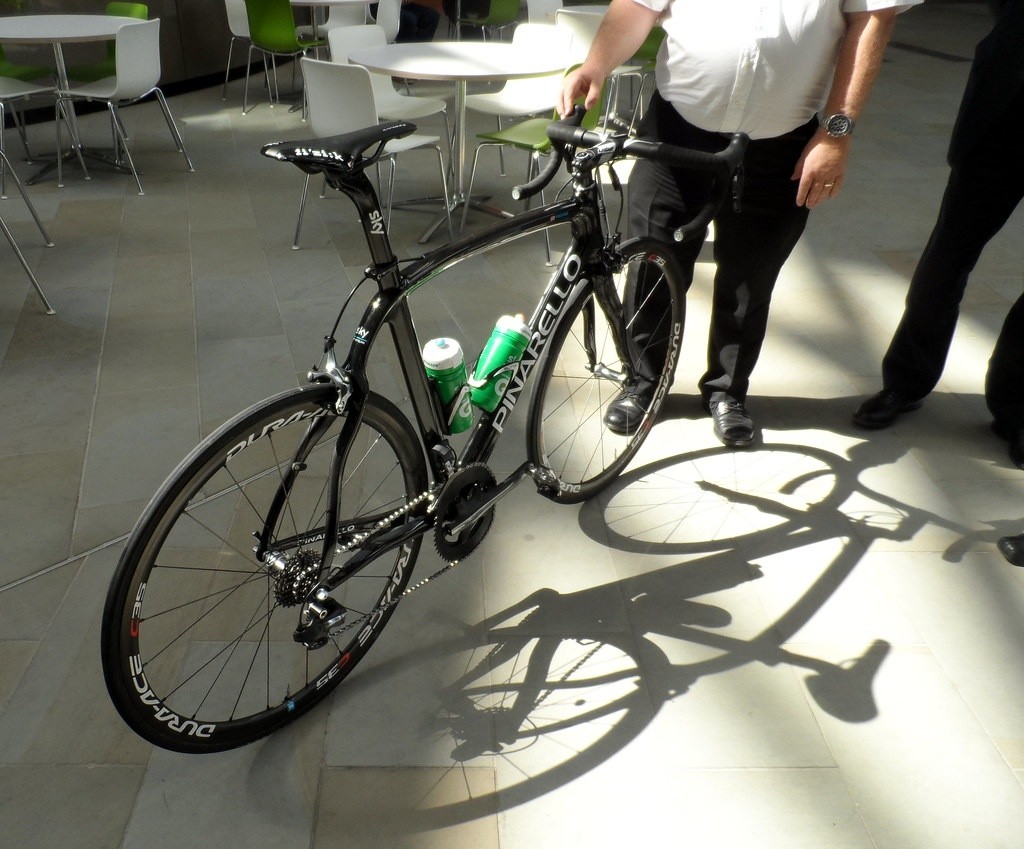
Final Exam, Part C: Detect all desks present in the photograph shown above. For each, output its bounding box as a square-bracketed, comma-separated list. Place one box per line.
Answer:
[0, 15, 149, 187]
[348, 42, 572, 243]
[563, 5, 611, 14]
[288, 0, 375, 113]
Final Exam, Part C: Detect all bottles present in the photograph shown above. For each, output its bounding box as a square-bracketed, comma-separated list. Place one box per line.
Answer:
[423, 337, 474, 434]
[469, 314, 532, 413]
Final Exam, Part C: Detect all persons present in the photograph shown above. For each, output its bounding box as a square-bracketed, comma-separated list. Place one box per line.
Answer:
[556, 0, 926, 448]
[850, 0, 1024, 469]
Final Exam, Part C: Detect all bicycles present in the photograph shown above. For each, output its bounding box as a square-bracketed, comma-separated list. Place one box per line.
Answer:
[97, 104, 752, 756]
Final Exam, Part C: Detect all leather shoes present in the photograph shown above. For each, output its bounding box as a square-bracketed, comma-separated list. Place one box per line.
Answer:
[603, 390, 648, 434]
[990, 420, 1024, 469]
[852, 390, 923, 431]
[997, 532, 1024, 567]
[708, 400, 755, 447]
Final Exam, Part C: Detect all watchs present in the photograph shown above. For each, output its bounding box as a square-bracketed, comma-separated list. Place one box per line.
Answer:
[816, 112, 856, 138]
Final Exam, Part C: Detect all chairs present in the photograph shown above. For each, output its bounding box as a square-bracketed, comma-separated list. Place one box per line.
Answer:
[459, 62, 612, 267]
[242, 0, 330, 122]
[61, 2, 184, 162]
[443, 20, 604, 222]
[0, 76, 91, 200]
[0, 44, 81, 164]
[0, 102, 56, 314]
[376, 1, 410, 97]
[222, 0, 295, 109]
[448, 0, 519, 42]
[291, 55, 455, 251]
[294, 0, 367, 60]
[53, 17, 195, 196]
[320, 25, 459, 229]
[367, 0, 376, 25]
[552, 5, 667, 139]
